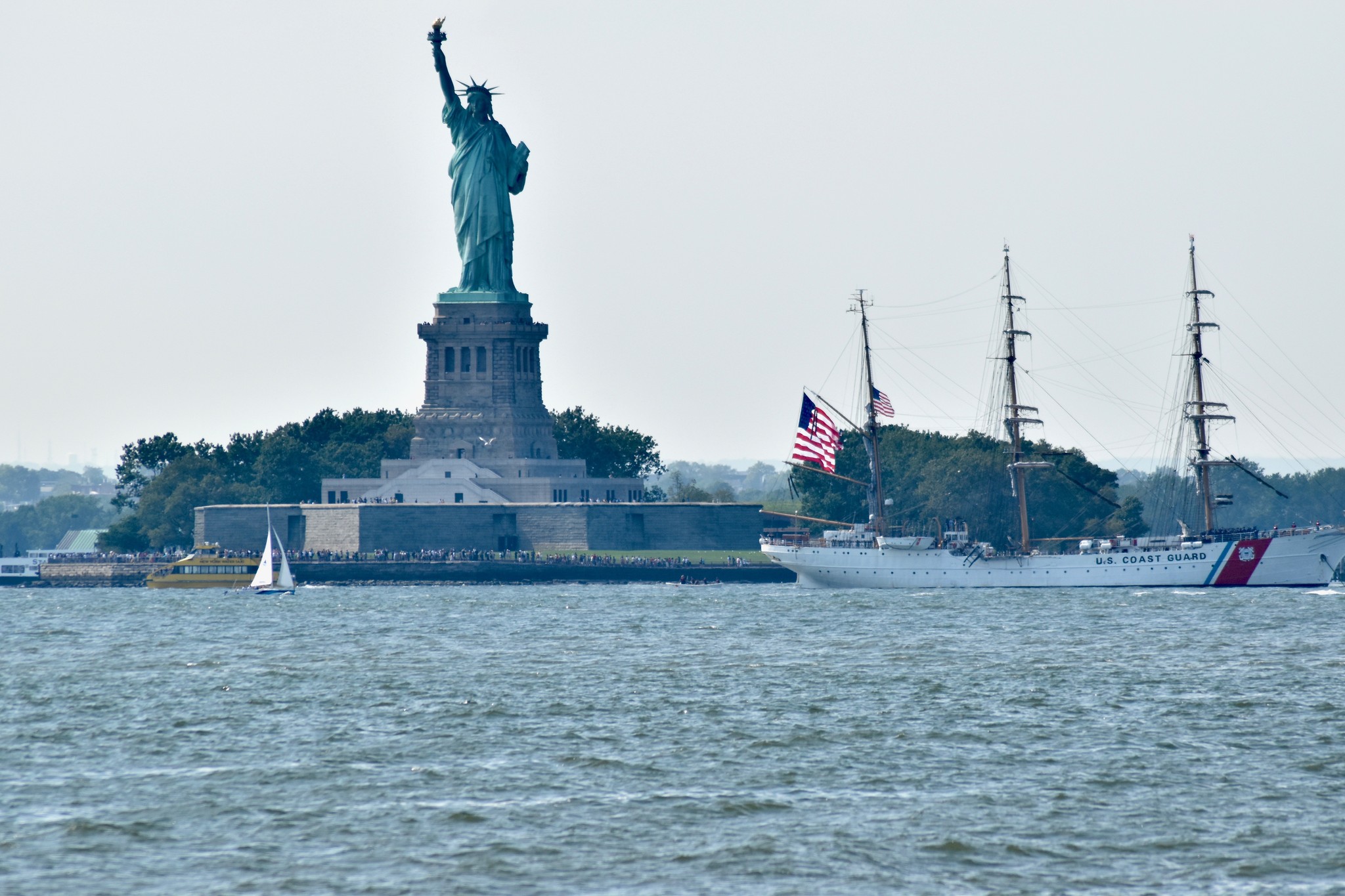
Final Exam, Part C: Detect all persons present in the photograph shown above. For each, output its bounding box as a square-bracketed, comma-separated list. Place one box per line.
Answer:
[759, 533, 774, 546]
[235, 584, 265, 591]
[680, 575, 720, 585]
[1199, 526, 1266, 543]
[1291, 522, 1297, 536]
[430, 42, 528, 292]
[49, 550, 186, 563]
[1273, 525, 1278, 537]
[1316, 521, 1320, 531]
[727, 555, 751, 567]
[942, 516, 973, 555]
[220, 548, 705, 567]
[579, 496, 648, 502]
[298, 496, 463, 505]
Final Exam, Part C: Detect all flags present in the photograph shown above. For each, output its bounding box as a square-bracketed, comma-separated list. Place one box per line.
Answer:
[792, 391, 847, 475]
[872, 386, 895, 418]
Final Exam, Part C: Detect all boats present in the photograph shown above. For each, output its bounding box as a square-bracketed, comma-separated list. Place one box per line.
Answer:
[876, 535, 935, 550]
[142, 541, 299, 590]
[665, 582, 724, 589]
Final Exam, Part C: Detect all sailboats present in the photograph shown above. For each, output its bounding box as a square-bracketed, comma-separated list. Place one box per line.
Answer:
[758, 232, 1345, 590]
[249, 500, 296, 596]
